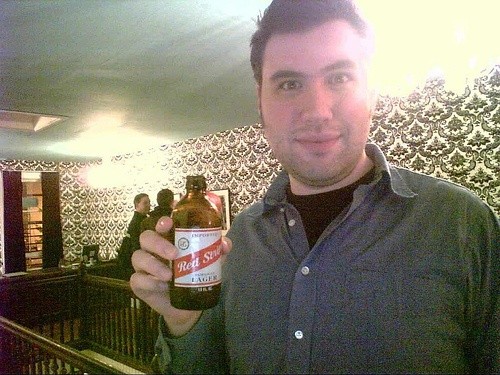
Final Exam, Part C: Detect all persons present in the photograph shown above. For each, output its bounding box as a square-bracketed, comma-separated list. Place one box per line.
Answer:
[118, 193, 152, 280]
[126, 0, 500, 374]
[139, 188, 176, 245]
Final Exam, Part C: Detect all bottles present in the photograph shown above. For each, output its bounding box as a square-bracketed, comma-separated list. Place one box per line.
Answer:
[167, 175, 223, 310]
